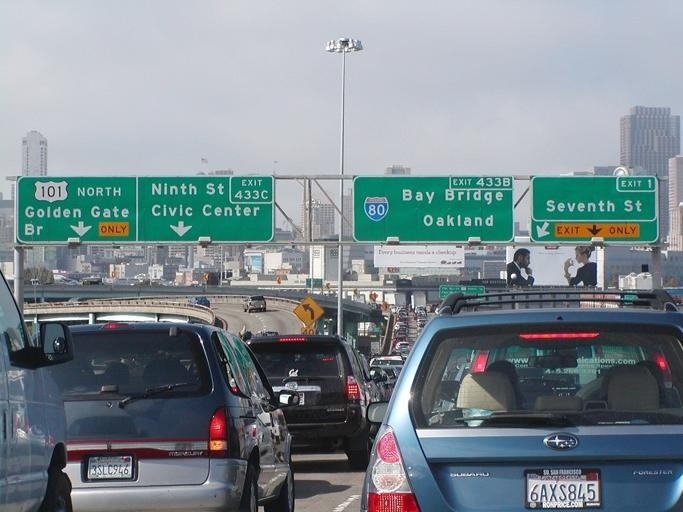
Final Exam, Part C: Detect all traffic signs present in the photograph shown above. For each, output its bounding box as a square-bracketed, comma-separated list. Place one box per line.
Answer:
[137, 177, 274, 243]
[531, 177, 658, 242]
[355, 178, 514, 244]
[17, 177, 134, 241]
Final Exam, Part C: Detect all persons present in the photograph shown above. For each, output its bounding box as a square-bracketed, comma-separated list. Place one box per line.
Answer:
[562, 245, 596, 288]
[506, 247, 535, 288]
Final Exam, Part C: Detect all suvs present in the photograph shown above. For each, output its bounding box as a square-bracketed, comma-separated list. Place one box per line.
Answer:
[240, 294, 266, 312]
[468, 344, 607, 431]
[241, 327, 388, 466]
[0, 266, 72, 511]
[361, 286, 682, 511]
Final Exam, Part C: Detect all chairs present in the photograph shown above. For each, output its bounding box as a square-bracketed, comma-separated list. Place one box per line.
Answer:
[607, 363, 660, 412]
[454, 372, 515, 410]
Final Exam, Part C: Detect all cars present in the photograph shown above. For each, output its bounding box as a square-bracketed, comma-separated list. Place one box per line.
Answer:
[187, 296, 210, 307]
[368, 303, 444, 389]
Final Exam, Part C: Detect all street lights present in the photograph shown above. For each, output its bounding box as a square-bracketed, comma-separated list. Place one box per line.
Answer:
[27, 274, 41, 346]
[325, 36, 366, 336]
[303, 199, 321, 289]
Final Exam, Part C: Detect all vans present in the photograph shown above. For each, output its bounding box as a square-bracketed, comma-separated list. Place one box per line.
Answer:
[55, 320, 294, 512]
